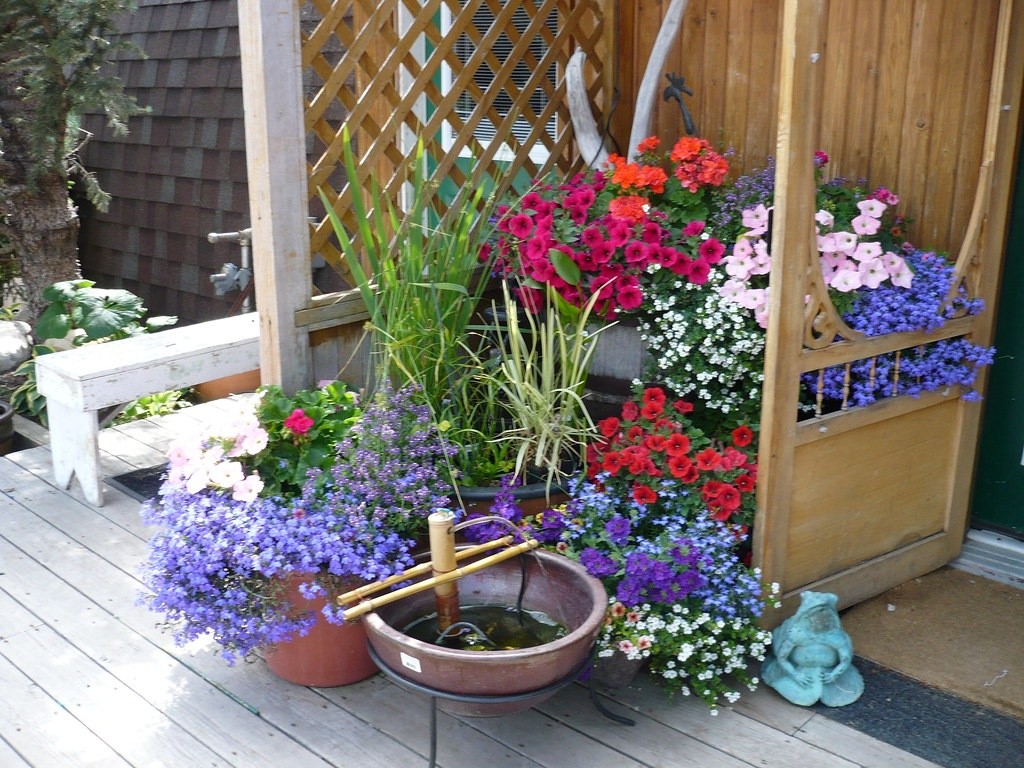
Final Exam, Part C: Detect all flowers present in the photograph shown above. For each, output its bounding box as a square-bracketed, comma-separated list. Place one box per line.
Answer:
[137, 122, 999, 718]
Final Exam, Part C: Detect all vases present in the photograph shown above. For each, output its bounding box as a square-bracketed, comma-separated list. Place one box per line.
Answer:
[261, 573, 382, 687]
[406, 443, 594, 554]
[362, 543, 609, 720]
[0, 399, 17, 457]
